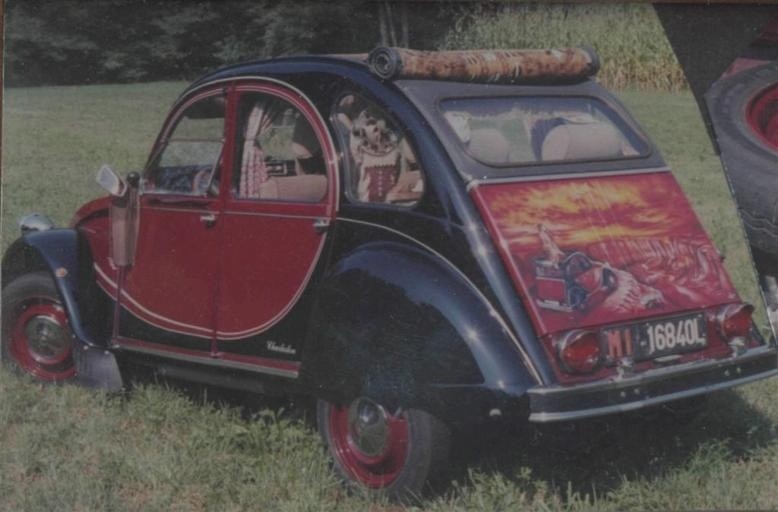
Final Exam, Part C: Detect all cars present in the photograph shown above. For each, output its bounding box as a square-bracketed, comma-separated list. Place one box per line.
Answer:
[0, 48, 778, 509]
[531, 249, 617, 314]
